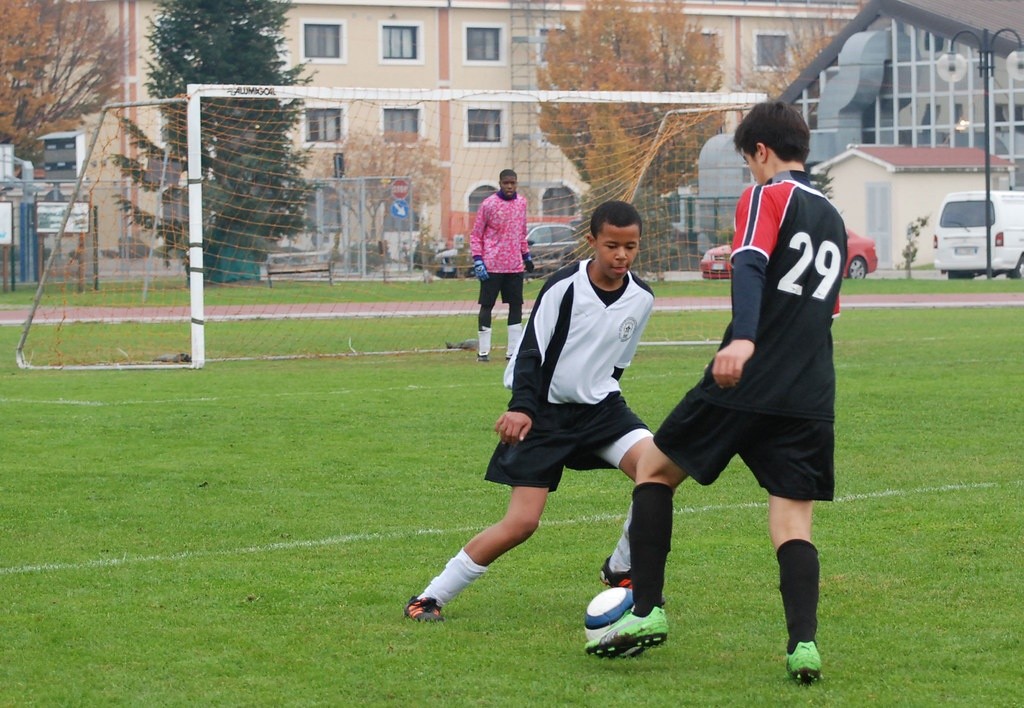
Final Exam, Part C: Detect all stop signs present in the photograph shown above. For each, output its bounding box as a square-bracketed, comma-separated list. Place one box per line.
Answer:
[390, 179, 410, 200]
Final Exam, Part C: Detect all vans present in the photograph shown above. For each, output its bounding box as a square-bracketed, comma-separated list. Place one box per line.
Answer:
[932, 191, 1024, 283]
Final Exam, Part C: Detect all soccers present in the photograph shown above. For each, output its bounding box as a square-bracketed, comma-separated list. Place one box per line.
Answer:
[584, 586, 646, 658]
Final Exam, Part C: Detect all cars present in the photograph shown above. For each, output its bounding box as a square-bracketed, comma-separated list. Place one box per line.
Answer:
[432, 222, 580, 280]
[698, 225, 878, 279]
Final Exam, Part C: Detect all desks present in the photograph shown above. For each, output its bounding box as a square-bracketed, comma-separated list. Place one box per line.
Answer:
[265, 247, 336, 288]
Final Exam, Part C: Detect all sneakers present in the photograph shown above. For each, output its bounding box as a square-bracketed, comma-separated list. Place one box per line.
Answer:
[786, 638, 825, 682]
[404, 596, 443, 622]
[599, 556, 665, 606]
[584, 606, 669, 658]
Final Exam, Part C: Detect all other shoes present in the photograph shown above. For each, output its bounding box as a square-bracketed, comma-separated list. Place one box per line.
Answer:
[477, 354, 489, 362]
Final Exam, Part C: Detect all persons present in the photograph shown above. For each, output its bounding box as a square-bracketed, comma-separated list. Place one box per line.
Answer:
[469, 170, 534, 362]
[583, 102, 847, 681]
[404, 202, 664, 622]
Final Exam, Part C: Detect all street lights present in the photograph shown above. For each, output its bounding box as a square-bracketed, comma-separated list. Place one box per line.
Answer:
[935, 24, 1024, 281]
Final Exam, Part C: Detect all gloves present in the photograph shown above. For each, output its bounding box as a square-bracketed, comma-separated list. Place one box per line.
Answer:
[474, 260, 489, 281]
[522, 253, 534, 273]
